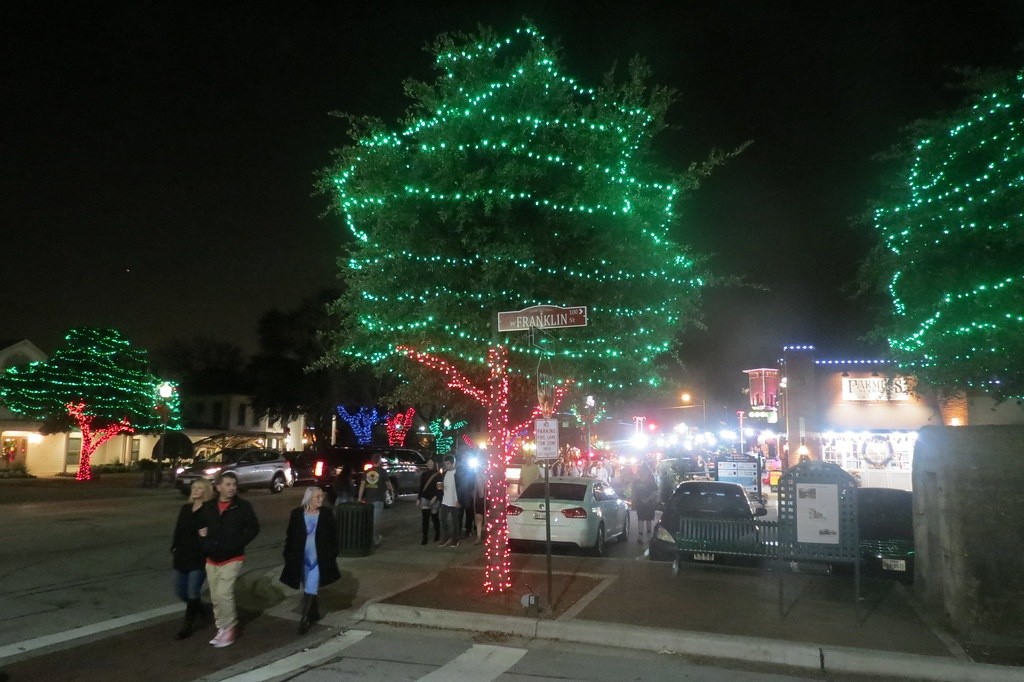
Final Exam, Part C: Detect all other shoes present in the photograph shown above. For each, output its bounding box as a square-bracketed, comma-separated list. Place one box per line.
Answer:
[439, 537, 452, 548]
[464, 530, 472, 537]
[638, 536, 643, 542]
[451, 540, 459, 548]
[209, 628, 224, 644]
[474, 538, 482, 545]
[216, 626, 237, 647]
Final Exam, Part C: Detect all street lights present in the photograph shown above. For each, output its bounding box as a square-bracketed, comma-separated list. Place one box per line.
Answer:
[682, 394, 706, 426]
[157, 381, 173, 480]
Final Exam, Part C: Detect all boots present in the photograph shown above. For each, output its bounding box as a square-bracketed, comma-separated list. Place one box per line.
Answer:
[421, 509, 430, 545]
[431, 512, 440, 541]
[298, 593, 316, 635]
[174, 600, 198, 640]
[313, 603, 320, 622]
[198, 601, 213, 624]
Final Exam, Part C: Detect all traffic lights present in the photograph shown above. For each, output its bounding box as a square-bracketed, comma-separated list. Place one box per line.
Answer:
[647, 420, 658, 435]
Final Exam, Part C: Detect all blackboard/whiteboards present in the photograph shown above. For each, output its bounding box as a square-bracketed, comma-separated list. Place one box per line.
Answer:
[534, 418, 559, 459]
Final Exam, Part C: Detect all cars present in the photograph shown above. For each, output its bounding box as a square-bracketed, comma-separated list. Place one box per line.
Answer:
[506, 477, 630, 557]
[830, 488, 914, 584]
[175, 447, 319, 493]
[649, 481, 768, 567]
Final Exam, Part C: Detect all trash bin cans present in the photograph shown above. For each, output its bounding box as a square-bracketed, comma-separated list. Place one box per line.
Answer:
[335, 501, 375, 557]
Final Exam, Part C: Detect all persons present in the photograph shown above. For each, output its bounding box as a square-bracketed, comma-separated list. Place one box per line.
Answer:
[358, 452, 395, 545]
[170, 477, 208, 641]
[279, 486, 341, 635]
[196, 469, 261, 649]
[418, 449, 487, 546]
[517, 441, 737, 545]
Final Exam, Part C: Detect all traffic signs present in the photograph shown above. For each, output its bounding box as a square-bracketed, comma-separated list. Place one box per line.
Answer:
[498, 305, 587, 332]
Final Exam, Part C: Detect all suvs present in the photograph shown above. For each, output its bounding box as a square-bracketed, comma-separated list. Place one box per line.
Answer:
[314, 447, 427, 510]
